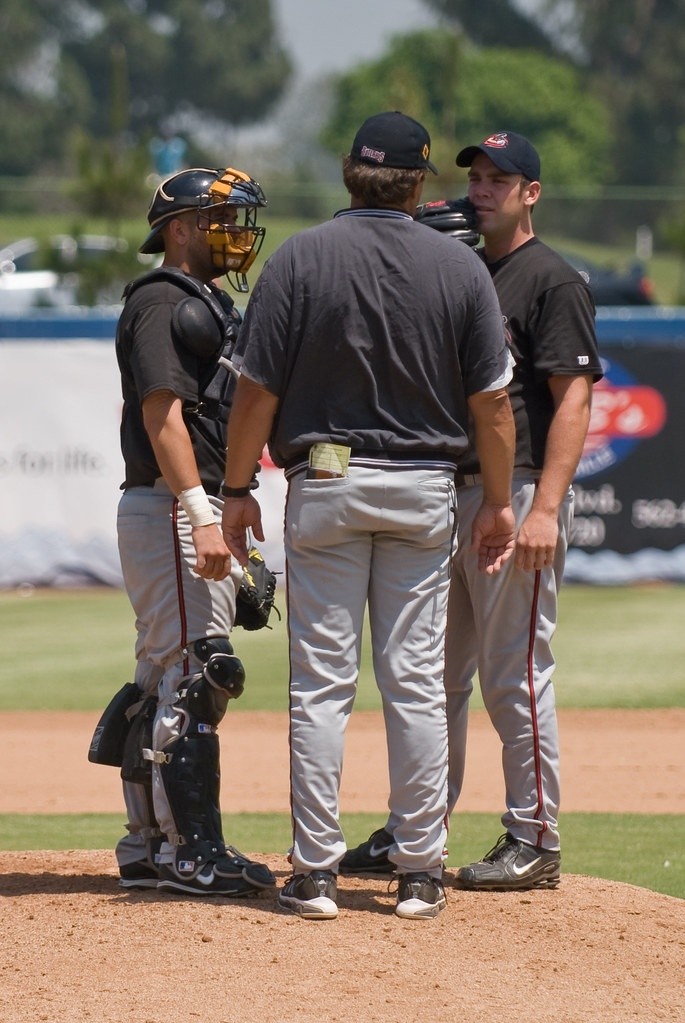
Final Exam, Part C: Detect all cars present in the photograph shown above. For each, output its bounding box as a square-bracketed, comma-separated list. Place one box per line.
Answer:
[549, 247, 660, 307]
[0, 232, 219, 310]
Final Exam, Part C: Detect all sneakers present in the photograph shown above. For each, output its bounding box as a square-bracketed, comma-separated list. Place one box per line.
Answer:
[339, 828, 398, 873]
[277, 870, 338, 918]
[157, 853, 275, 897]
[453, 832, 561, 890]
[388, 871, 447, 920]
[119, 858, 161, 889]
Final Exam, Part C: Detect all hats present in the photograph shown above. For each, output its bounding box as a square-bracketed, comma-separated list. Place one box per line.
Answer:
[351, 110, 439, 175]
[456, 131, 541, 181]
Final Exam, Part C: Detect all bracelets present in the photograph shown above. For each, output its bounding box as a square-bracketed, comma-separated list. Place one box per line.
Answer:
[221, 483, 251, 498]
[177, 484, 217, 527]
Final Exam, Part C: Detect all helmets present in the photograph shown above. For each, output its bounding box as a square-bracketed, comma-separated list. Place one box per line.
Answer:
[138, 166, 223, 254]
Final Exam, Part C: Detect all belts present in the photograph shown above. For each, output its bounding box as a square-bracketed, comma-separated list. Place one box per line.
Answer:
[120, 477, 220, 498]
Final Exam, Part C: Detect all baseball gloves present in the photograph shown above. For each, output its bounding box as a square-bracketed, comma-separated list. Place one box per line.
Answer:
[234, 546, 284, 631]
[414, 196, 481, 247]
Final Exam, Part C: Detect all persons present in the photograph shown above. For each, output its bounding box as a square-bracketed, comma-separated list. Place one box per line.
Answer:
[225, 123, 517, 919]
[337, 130, 605, 895]
[114, 166, 278, 894]
